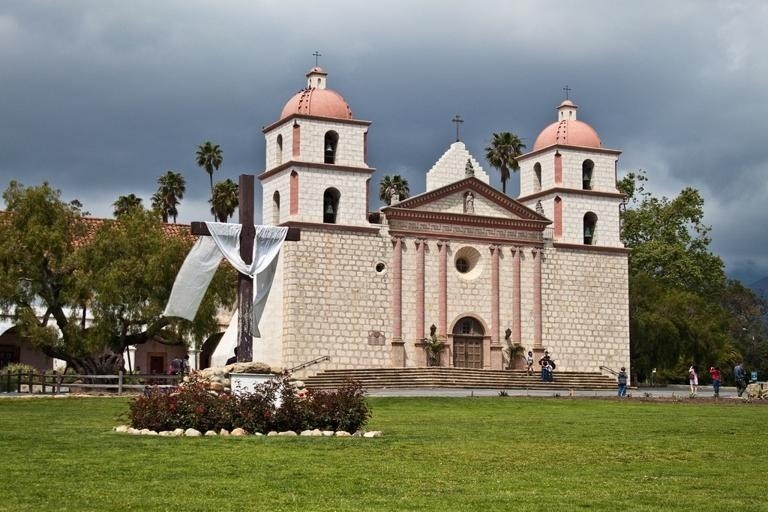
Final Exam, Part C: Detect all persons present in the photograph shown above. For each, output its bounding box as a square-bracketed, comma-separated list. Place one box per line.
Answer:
[224, 346, 238, 378]
[688, 365, 698, 394]
[391, 185, 398, 196]
[169, 355, 191, 385]
[541, 351, 553, 381]
[710, 366, 720, 397]
[466, 192, 474, 214]
[734, 361, 746, 397]
[465, 159, 474, 174]
[617, 367, 628, 396]
[132, 366, 145, 390]
[536, 199, 543, 210]
[526, 351, 533, 375]
